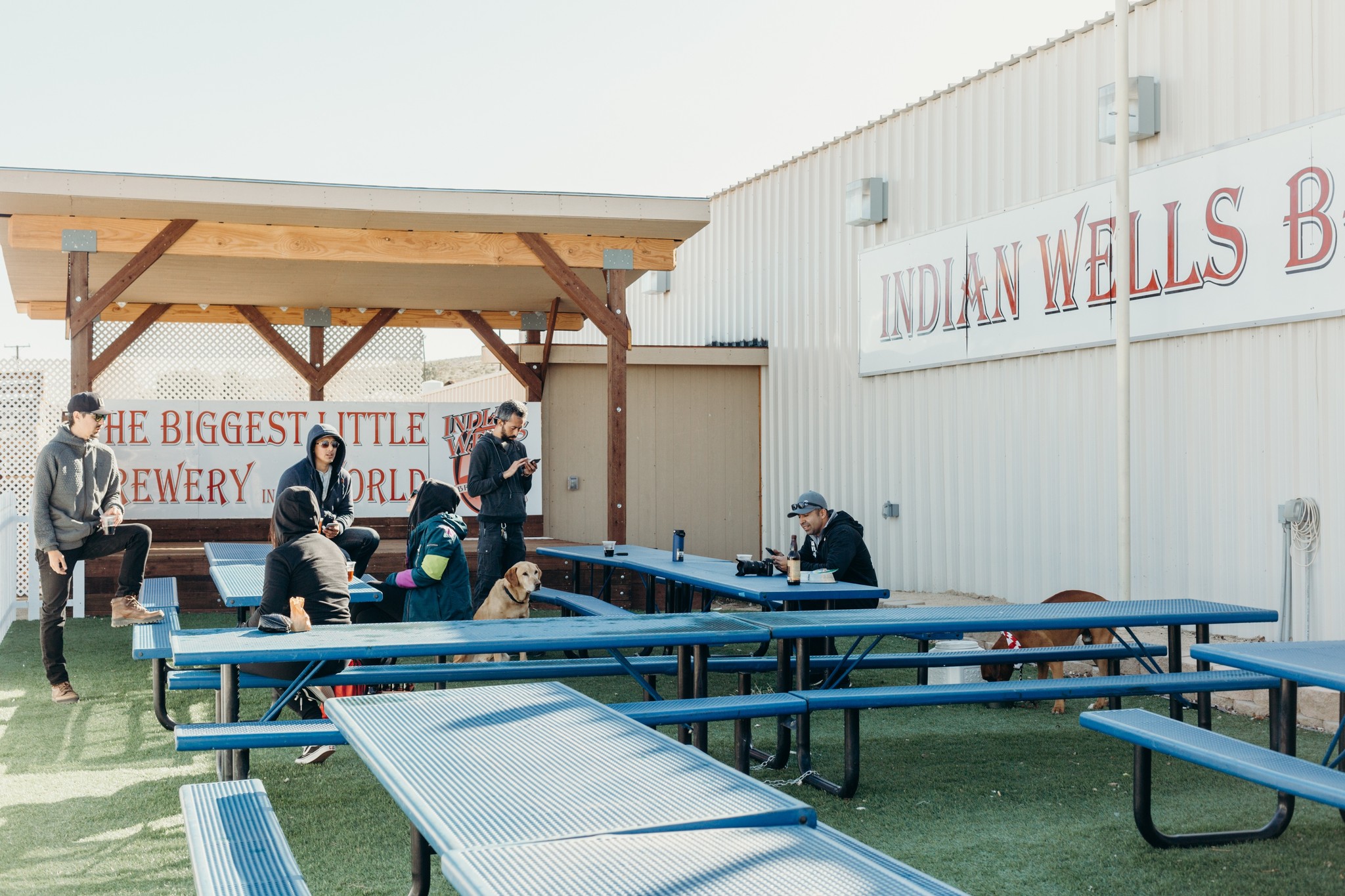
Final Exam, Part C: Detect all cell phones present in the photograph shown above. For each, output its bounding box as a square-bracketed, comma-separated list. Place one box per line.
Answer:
[521, 459, 541, 470]
[766, 547, 776, 556]
[323, 523, 338, 533]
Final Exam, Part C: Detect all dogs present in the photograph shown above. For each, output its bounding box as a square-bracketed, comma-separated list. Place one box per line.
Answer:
[980, 590, 1118, 716]
[454, 561, 542, 661]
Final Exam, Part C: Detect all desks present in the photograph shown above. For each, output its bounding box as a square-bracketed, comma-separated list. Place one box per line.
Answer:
[325, 681, 817, 896]
[442, 818, 970, 896]
[536, 545, 732, 654]
[622, 559, 890, 682]
[1190, 640, 1345, 831]
[168, 610, 771, 773]
[727, 598, 1278, 795]
[203, 542, 383, 627]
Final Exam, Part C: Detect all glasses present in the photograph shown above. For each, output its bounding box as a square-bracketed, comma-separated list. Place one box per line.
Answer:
[493, 416, 503, 425]
[315, 440, 339, 448]
[80, 411, 107, 421]
[410, 489, 418, 499]
[791, 501, 823, 511]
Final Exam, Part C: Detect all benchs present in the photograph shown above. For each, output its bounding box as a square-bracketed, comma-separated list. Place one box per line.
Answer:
[131, 577, 1345, 896]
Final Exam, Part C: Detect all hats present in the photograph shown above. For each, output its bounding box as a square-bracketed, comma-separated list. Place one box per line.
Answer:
[314, 433, 341, 442]
[787, 490, 828, 518]
[68, 391, 117, 415]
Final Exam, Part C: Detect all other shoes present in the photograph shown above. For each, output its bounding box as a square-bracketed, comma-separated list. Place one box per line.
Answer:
[506, 651, 544, 657]
[810, 674, 852, 689]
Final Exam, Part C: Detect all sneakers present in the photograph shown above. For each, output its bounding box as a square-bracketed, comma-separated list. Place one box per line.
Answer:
[51, 681, 79, 704]
[294, 685, 335, 711]
[110, 594, 165, 628]
[295, 745, 335, 764]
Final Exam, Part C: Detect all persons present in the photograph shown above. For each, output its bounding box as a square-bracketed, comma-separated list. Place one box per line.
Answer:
[467, 399, 544, 657]
[349, 478, 472, 694]
[772, 489, 879, 688]
[275, 424, 380, 579]
[33, 390, 165, 706]
[238, 486, 351, 764]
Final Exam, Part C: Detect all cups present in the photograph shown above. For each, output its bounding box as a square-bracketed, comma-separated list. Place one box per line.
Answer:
[736, 554, 752, 563]
[347, 562, 356, 582]
[99, 515, 116, 535]
[602, 541, 616, 557]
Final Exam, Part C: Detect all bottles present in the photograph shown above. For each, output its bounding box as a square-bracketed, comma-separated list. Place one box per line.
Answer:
[672, 530, 685, 561]
[787, 535, 800, 585]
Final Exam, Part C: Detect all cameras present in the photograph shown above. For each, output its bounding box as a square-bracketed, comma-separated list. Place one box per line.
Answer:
[735, 558, 773, 576]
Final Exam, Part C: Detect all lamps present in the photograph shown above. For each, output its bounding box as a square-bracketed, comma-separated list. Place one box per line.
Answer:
[641, 270, 670, 294]
[1098, 75, 1163, 144]
[846, 176, 888, 228]
